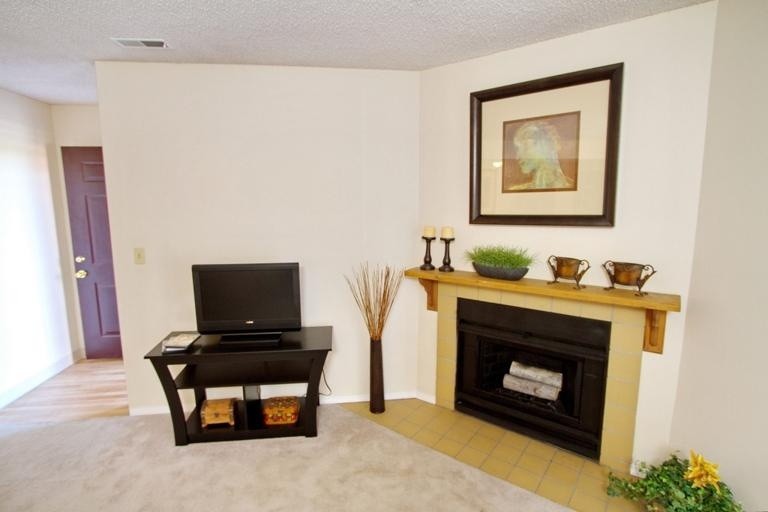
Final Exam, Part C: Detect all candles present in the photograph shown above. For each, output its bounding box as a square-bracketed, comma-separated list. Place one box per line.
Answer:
[423, 225, 454, 239]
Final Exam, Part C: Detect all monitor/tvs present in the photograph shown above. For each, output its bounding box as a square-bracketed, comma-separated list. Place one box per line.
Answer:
[192, 263, 301, 344]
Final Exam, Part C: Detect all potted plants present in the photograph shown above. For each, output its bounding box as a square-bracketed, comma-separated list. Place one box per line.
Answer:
[467, 245, 535, 281]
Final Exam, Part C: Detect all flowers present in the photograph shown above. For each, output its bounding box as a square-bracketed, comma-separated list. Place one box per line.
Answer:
[605, 447, 745, 512]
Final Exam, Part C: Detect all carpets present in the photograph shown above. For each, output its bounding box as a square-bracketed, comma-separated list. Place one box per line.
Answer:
[1, 399, 583, 511]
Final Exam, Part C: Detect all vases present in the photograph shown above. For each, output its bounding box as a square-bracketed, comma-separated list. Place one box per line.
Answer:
[369, 336, 386, 414]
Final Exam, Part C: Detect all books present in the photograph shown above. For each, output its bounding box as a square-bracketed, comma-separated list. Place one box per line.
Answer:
[159, 331, 200, 355]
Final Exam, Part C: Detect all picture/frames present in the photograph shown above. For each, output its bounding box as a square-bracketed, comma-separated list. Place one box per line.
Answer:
[467, 60, 624, 227]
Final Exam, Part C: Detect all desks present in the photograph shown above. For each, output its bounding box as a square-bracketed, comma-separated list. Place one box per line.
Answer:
[144, 325, 334, 446]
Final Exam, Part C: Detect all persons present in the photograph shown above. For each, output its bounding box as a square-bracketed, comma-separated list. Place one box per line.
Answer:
[505, 120, 574, 190]
